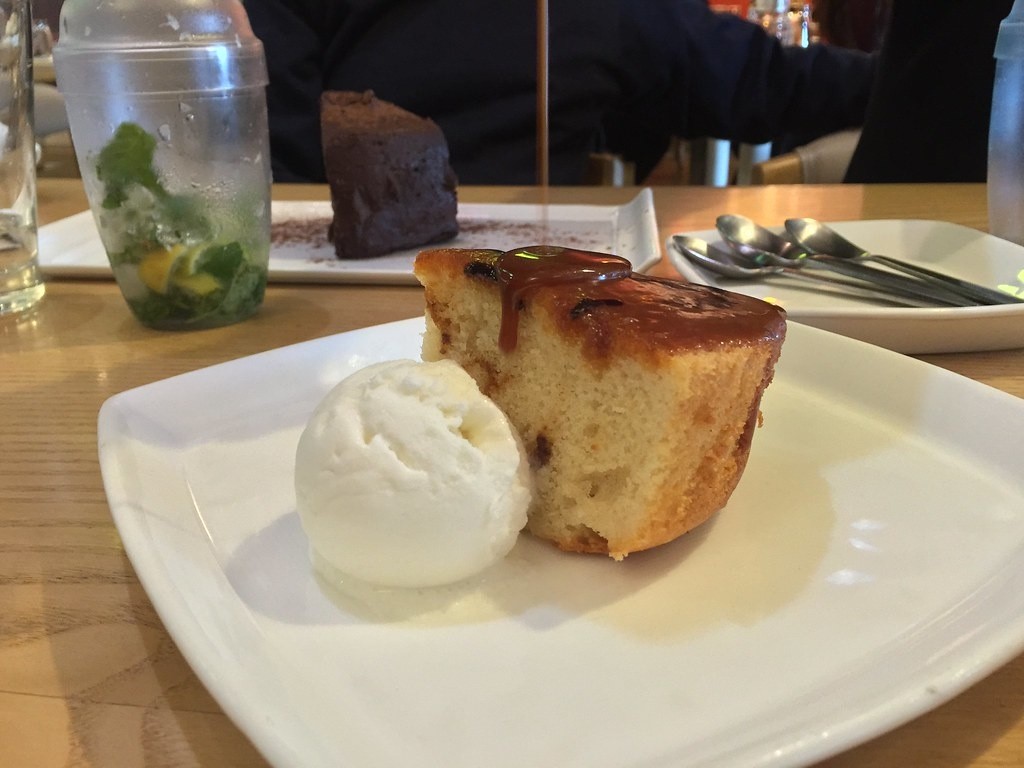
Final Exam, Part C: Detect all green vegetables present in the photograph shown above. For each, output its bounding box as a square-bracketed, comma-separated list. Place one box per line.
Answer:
[94, 121, 267, 329]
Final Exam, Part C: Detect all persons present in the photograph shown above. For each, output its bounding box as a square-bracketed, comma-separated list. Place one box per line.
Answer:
[235, 0, 883, 183]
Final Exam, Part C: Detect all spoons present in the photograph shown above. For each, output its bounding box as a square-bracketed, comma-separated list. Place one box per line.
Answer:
[673, 212, 1024, 314]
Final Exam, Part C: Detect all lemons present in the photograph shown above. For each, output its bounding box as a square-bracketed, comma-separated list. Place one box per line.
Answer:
[138, 245, 187, 296]
[182, 238, 219, 277]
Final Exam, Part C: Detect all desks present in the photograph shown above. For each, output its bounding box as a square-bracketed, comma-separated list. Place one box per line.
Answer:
[0, 181, 1024, 768]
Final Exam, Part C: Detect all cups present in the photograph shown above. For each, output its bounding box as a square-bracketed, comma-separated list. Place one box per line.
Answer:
[52, 0, 268, 331]
[0, 0, 46, 316]
[985, 1, 1024, 243]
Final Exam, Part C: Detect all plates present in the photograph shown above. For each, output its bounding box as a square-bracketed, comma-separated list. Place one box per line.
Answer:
[37, 186, 662, 284]
[666, 219, 1023, 354]
[97, 313, 1024, 768]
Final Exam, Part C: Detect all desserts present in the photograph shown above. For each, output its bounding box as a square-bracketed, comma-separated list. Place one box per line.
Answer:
[296, 357, 530, 590]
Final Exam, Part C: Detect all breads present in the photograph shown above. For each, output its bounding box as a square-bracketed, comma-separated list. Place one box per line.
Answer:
[319, 89, 460, 262]
[410, 242, 787, 557]
